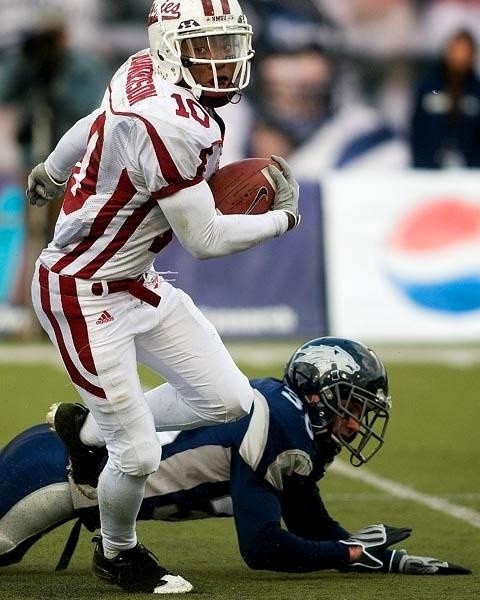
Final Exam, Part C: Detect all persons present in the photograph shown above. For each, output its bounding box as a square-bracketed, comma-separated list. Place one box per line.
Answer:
[20, 1, 304, 595]
[0, 334, 479, 579]
[1, 0, 480, 167]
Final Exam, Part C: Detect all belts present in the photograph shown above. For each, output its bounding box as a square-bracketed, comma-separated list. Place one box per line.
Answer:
[93, 273, 161, 317]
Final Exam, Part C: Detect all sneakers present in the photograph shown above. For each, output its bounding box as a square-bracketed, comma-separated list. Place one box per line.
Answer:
[91, 535, 194, 593]
[46, 402, 107, 500]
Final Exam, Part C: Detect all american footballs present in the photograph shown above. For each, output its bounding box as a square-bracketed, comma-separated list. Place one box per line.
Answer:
[207, 158, 280, 215]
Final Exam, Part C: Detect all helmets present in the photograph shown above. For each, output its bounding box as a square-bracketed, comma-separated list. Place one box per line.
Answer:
[147, 0, 256, 104]
[285, 336, 392, 468]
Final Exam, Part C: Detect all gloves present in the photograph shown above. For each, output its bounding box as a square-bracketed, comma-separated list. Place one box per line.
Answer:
[268, 154, 302, 231]
[26, 162, 69, 207]
[339, 524, 473, 575]
